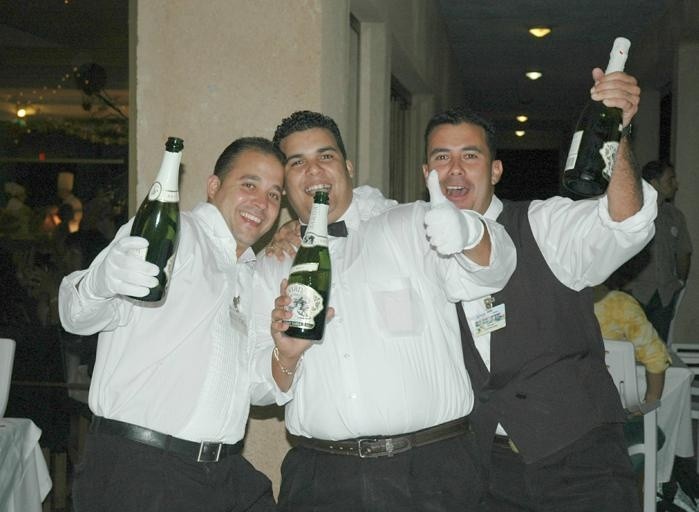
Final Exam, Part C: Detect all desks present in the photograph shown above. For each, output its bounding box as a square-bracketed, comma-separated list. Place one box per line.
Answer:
[0, 417, 54, 512]
[635, 365, 694, 501]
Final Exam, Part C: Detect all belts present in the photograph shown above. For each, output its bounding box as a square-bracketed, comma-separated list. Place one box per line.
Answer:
[283, 412, 473, 461]
[87, 413, 246, 466]
[491, 433, 511, 450]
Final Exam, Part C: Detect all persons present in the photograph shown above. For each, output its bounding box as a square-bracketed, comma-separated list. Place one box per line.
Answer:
[6, 188, 27, 212]
[421, 68, 658, 512]
[247, 111, 516, 512]
[607, 161, 692, 344]
[593, 278, 674, 475]
[50, 187, 82, 233]
[58, 138, 335, 512]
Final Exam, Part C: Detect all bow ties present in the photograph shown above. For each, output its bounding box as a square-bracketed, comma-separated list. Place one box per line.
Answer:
[299, 220, 350, 240]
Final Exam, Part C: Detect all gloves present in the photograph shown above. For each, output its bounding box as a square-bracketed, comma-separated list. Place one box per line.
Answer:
[423, 168, 485, 257]
[79, 236, 161, 301]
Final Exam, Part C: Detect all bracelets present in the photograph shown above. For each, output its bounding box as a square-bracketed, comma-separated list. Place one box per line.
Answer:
[678, 280, 684, 288]
[273, 347, 302, 376]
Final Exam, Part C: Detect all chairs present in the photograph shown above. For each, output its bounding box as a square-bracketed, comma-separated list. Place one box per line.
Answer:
[672, 344, 699, 419]
[0, 338, 17, 417]
[603, 339, 660, 512]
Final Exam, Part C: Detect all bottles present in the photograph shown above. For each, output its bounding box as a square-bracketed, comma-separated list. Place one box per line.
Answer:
[278, 190, 331, 342]
[118, 134, 186, 303]
[562, 33, 634, 196]
